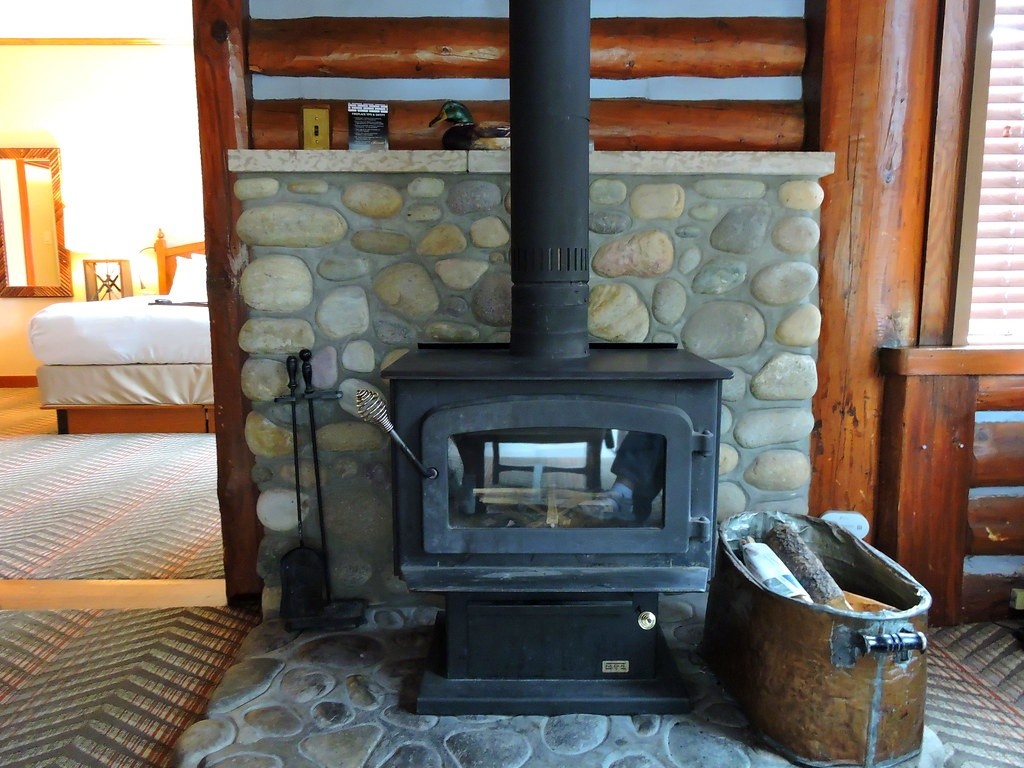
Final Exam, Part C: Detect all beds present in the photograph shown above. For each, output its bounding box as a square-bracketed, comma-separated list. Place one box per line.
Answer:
[28, 227, 216, 435]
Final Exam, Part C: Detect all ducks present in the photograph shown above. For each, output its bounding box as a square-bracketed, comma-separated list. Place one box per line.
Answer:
[428, 100, 511, 150]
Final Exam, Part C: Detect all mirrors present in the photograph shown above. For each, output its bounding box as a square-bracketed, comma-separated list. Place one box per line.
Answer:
[0, 147, 79, 298]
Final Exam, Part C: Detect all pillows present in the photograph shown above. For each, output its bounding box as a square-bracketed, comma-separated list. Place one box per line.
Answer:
[169, 252, 209, 303]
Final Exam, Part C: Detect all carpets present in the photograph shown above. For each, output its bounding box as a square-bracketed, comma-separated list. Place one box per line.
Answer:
[0, 386, 225, 579]
[0, 606, 262, 768]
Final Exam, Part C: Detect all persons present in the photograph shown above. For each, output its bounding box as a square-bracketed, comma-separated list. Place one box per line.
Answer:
[579, 431, 667, 522]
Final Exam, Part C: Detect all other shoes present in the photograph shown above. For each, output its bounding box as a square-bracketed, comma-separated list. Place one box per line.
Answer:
[574, 492, 637, 522]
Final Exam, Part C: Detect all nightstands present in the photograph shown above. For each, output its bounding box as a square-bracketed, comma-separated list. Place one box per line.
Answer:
[83, 259, 133, 302]
